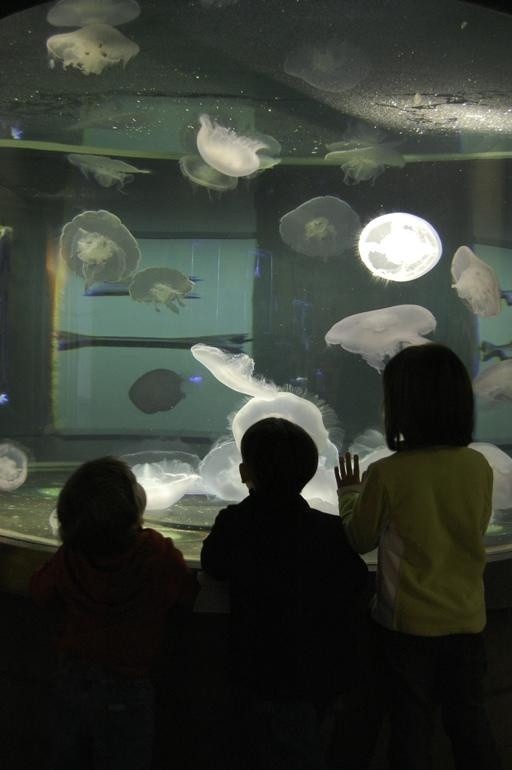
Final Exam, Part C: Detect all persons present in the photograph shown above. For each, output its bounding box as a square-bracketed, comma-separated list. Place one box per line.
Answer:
[26, 451, 195, 767]
[196, 416, 375, 768]
[333, 339, 501, 770]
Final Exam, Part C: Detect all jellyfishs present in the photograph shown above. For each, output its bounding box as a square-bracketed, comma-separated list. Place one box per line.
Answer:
[0, 0, 512, 538]
